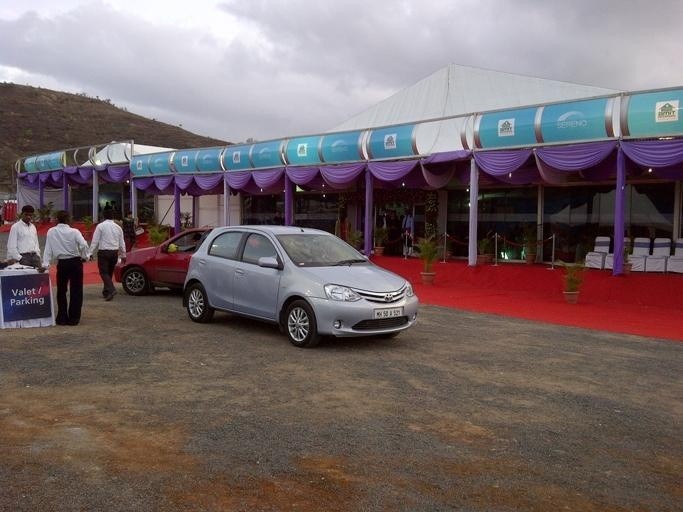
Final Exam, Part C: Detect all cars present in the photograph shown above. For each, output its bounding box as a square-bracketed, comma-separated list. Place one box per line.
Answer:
[114, 224, 214, 295]
[182, 224, 420, 349]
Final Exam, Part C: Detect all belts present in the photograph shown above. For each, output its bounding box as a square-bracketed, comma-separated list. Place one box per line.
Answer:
[19, 252, 37, 255]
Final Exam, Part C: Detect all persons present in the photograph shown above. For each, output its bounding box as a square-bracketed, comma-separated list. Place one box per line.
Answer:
[97, 199, 121, 219]
[36, 209, 90, 326]
[0, 258, 16, 269]
[86, 208, 126, 300]
[399, 207, 415, 257]
[5, 203, 41, 268]
[120, 210, 138, 243]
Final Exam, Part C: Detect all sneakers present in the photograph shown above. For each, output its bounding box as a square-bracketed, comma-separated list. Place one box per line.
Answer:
[102, 290, 117, 302]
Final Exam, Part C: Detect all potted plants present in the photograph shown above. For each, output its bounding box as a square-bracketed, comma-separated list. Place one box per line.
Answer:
[36, 201, 54, 226]
[414, 234, 493, 287]
[525, 236, 538, 264]
[80, 215, 93, 240]
[372, 227, 388, 256]
[558, 262, 584, 305]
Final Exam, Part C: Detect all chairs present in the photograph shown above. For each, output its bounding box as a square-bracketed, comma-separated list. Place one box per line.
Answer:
[583, 234, 682, 278]
